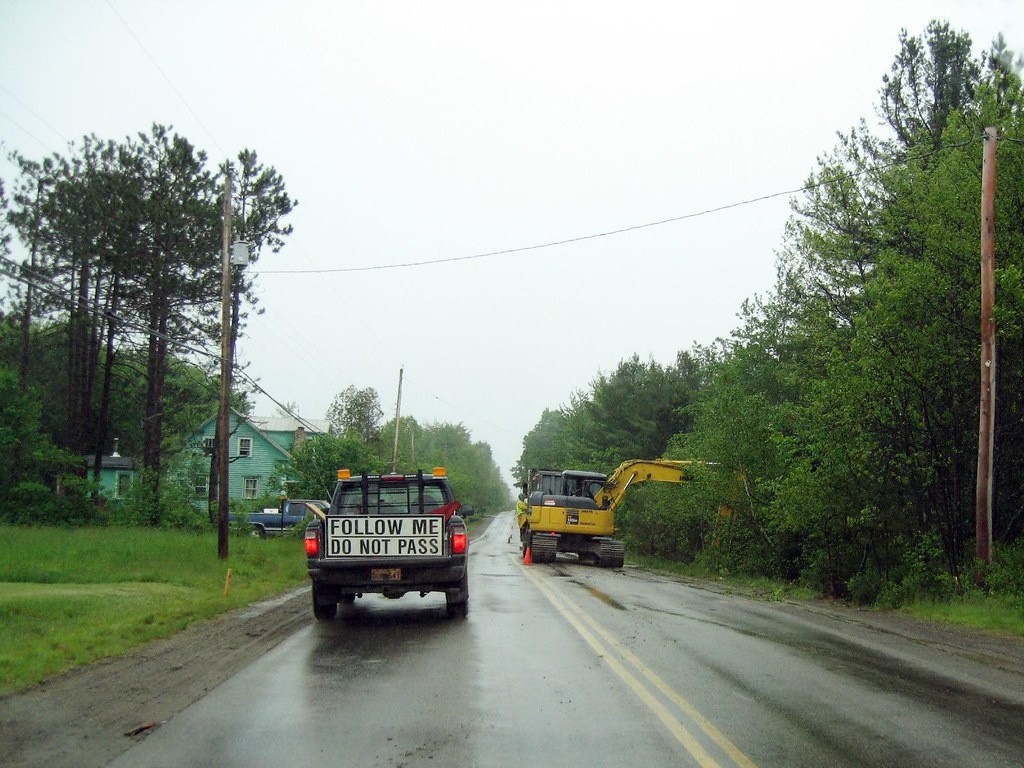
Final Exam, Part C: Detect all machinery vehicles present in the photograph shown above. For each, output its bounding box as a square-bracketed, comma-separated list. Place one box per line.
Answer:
[518, 458, 745, 569]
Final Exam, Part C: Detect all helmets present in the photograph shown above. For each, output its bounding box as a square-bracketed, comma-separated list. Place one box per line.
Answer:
[518, 494, 525, 498]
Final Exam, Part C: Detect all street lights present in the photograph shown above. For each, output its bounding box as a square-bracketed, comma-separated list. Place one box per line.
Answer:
[278, 490, 287, 533]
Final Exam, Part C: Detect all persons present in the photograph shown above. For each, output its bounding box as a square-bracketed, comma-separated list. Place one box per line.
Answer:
[516, 493, 529, 552]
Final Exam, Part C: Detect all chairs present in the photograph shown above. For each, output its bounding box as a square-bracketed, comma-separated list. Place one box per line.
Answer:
[410, 494, 438, 516]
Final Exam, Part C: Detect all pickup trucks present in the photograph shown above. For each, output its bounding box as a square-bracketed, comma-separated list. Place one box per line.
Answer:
[304, 466, 475, 622]
[227, 498, 332, 540]
